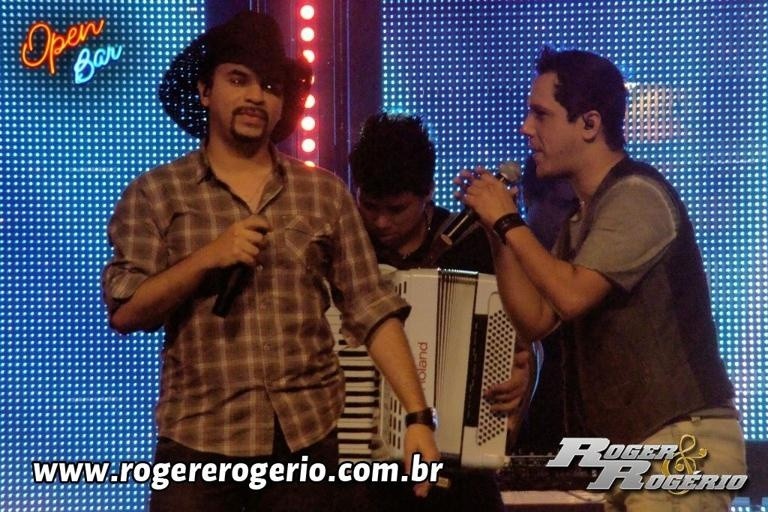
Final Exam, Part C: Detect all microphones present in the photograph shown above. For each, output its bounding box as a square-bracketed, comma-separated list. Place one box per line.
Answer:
[441, 160, 522, 246]
[210, 215, 268, 318]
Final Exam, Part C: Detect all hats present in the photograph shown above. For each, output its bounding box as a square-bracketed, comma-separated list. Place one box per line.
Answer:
[158, 11, 313, 146]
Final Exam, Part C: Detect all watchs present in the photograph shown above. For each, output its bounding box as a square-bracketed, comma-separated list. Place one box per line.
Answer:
[491, 213, 526, 246]
[403, 405, 438, 433]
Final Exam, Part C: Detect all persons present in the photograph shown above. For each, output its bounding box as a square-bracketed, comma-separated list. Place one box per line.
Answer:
[452, 41, 749, 512]
[97, 10, 442, 511]
[346, 108, 544, 510]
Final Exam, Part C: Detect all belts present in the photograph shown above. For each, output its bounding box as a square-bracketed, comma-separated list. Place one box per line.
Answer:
[681, 405, 738, 416]
